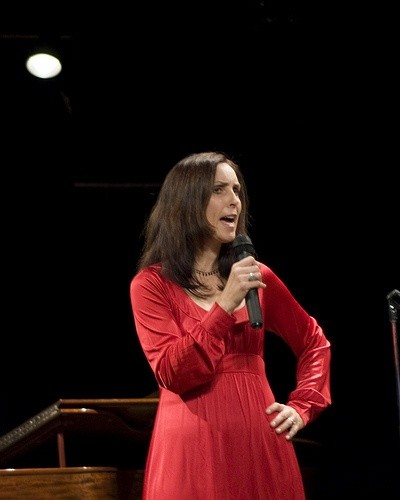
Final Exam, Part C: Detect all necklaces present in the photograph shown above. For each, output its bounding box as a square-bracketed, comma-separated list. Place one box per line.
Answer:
[192, 269, 219, 276]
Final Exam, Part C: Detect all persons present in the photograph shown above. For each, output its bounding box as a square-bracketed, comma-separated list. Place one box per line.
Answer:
[130, 152, 330, 500]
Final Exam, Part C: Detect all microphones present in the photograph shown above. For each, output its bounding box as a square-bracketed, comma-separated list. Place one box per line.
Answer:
[232, 233, 263, 329]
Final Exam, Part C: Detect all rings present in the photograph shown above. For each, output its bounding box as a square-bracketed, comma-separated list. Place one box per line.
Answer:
[288, 418, 295, 425]
[249, 273, 257, 281]
